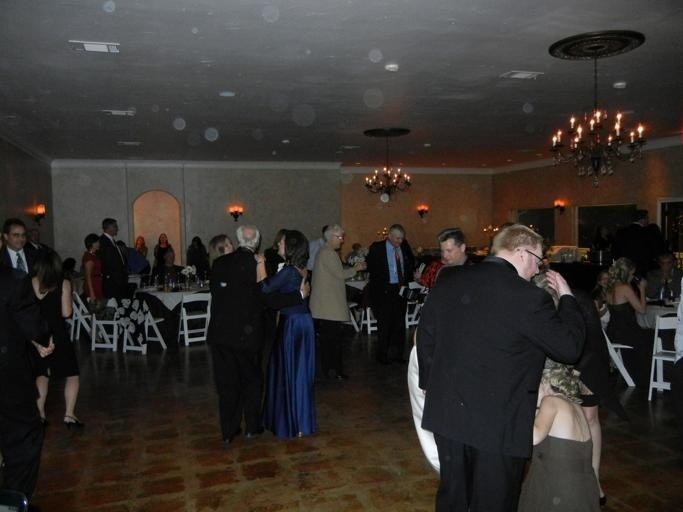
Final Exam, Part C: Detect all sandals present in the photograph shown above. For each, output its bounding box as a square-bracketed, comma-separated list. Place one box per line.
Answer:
[63, 413, 83, 428]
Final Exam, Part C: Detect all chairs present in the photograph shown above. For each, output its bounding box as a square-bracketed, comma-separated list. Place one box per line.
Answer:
[598, 324, 638, 388]
[177, 293, 212, 346]
[648, 316, 678, 403]
[346, 302, 363, 333]
[85, 297, 122, 352]
[118, 297, 166, 354]
[359, 305, 378, 333]
[404, 301, 423, 329]
[68, 290, 93, 342]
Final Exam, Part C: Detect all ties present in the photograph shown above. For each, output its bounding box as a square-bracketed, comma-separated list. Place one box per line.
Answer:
[394, 247, 403, 281]
[14, 251, 25, 271]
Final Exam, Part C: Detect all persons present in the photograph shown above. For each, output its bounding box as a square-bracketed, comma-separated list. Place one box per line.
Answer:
[266, 230, 287, 279]
[437, 226, 482, 268]
[153, 233, 175, 287]
[115, 239, 151, 280]
[159, 252, 181, 289]
[305, 225, 329, 280]
[134, 236, 148, 259]
[31, 249, 86, 431]
[0, 267, 54, 512]
[82, 232, 103, 305]
[626, 209, 654, 274]
[646, 248, 683, 299]
[366, 224, 420, 363]
[515, 356, 602, 512]
[187, 236, 211, 281]
[209, 233, 234, 268]
[414, 224, 589, 512]
[98, 218, 128, 308]
[62, 257, 85, 300]
[210, 223, 310, 444]
[311, 224, 368, 380]
[0, 219, 40, 282]
[255, 229, 318, 439]
[594, 270, 611, 332]
[606, 257, 656, 389]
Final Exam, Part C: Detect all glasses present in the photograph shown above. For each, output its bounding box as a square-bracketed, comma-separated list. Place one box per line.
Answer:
[517, 245, 548, 268]
[334, 234, 343, 240]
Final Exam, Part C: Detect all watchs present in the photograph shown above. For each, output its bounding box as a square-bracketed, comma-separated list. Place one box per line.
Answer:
[257, 260, 265, 264]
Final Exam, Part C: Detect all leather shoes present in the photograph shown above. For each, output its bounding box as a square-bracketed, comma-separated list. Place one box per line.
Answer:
[242, 428, 264, 438]
[220, 431, 233, 443]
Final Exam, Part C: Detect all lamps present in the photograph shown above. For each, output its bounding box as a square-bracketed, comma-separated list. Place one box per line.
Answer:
[362, 126, 413, 206]
[417, 203, 430, 217]
[482, 224, 500, 234]
[374, 224, 390, 240]
[553, 199, 567, 215]
[34, 204, 47, 226]
[228, 205, 244, 221]
[547, 26, 646, 181]
